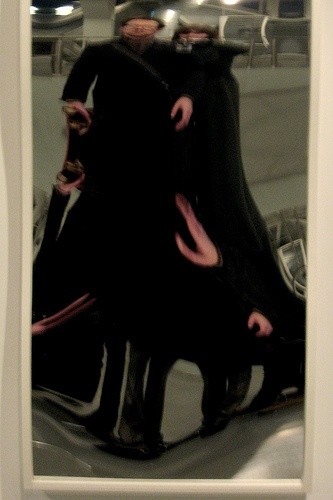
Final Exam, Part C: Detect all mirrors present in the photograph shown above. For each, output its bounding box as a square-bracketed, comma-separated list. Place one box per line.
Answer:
[15, 0, 322, 497]
